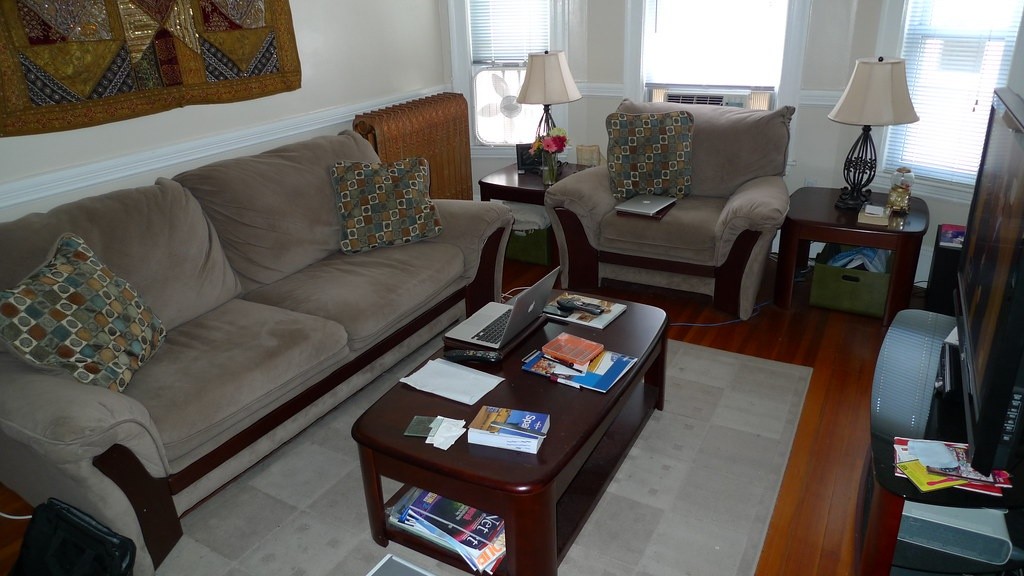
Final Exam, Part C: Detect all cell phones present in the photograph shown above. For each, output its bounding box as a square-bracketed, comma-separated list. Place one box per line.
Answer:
[543, 304, 575, 319]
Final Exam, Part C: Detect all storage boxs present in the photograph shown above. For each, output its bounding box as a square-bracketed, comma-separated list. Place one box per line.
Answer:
[808, 243, 894, 317]
[496, 210, 551, 266]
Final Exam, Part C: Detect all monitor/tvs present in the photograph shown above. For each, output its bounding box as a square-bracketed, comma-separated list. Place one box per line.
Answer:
[953, 86, 1024, 475]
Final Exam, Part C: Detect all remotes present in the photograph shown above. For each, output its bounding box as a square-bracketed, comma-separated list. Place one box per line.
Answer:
[444, 350, 503, 364]
[556, 298, 605, 315]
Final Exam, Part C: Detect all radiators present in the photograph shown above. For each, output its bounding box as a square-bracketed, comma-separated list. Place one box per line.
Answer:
[353, 90, 473, 200]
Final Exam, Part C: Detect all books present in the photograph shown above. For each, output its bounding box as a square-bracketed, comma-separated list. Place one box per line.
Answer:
[522, 331, 638, 392]
[468, 405, 551, 455]
[940, 224, 965, 247]
[389, 489, 506, 576]
[893, 436, 1012, 496]
[859, 202, 893, 225]
[545, 292, 627, 329]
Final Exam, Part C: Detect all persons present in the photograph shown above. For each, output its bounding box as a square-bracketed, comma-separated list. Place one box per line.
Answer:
[942, 231, 964, 243]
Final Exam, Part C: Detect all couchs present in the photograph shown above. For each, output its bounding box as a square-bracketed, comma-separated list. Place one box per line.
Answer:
[545, 98, 796, 320]
[0, 129, 513, 576]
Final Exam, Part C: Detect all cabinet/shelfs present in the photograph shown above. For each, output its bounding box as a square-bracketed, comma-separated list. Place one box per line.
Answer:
[946, 97, 1024, 468]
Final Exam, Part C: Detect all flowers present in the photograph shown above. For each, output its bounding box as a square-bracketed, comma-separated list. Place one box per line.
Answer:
[528, 128, 570, 153]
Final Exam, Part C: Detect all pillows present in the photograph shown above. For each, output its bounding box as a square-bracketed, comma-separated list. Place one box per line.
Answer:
[605, 111, 694, 201]
[330, 158, 443, 255]
[0, 230, 167, 393]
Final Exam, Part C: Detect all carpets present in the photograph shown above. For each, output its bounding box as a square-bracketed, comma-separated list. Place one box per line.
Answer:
[156, 311, 813, 576]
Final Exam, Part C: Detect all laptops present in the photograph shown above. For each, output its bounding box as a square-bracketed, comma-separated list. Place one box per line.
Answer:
[443, 265, 561, 351]
[615, 195, 678, 216]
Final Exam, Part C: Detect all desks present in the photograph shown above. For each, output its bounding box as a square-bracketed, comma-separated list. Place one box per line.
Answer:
[777, 187, 930, 325]
[478, 163, 594, 204]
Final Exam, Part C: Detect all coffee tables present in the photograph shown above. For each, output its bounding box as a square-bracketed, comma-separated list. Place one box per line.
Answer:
[351, 288, 666, 576]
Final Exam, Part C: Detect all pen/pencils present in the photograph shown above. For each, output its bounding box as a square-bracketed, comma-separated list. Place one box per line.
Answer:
[552, 356, 574, 367]
[541, 355, 584, 373]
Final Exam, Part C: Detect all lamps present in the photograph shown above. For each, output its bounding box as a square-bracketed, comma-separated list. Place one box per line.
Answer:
[516, 51, 583, 138]
[827, 55, 919, 208]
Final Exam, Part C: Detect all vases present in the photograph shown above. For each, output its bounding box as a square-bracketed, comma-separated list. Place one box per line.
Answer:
[542, 151, 558, 186]
